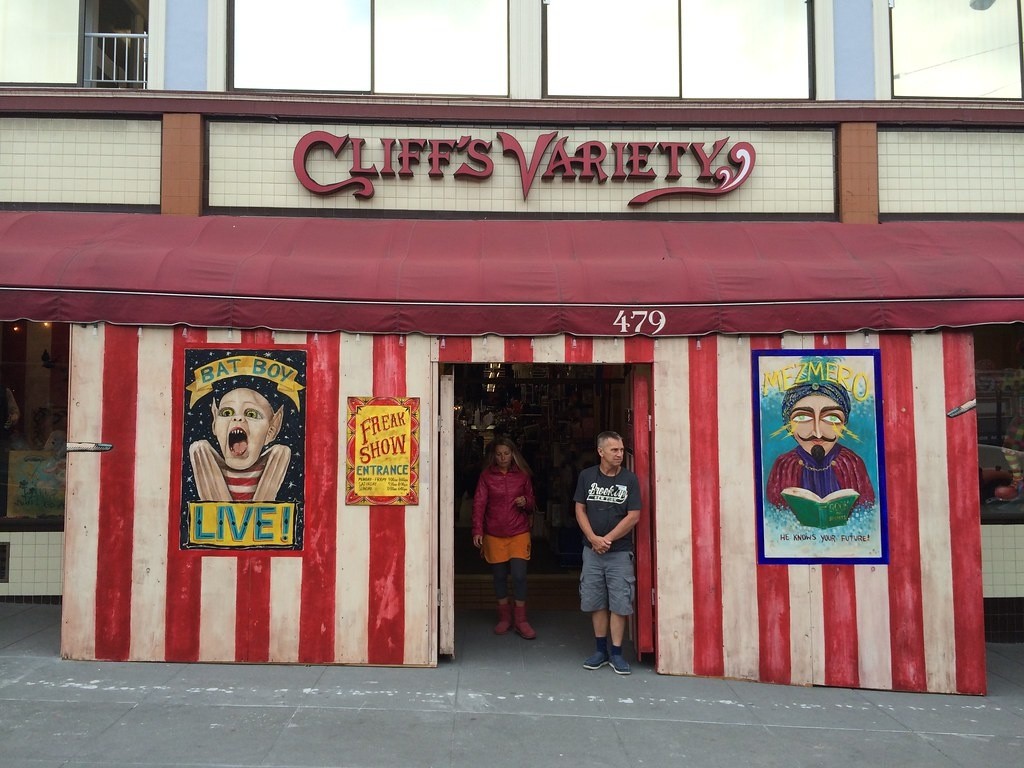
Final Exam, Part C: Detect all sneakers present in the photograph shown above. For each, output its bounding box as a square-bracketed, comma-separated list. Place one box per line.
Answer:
[583, 652, 609, 670]
[607, 657, 632, 674]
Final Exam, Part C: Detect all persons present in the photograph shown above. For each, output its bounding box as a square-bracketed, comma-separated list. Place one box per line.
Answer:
[572, 431, 642, 675]
[471, 436, 535, 640]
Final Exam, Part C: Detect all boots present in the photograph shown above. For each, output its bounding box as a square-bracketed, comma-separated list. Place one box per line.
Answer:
[513, 605, 535, 638]
[494, 604, 512, 634]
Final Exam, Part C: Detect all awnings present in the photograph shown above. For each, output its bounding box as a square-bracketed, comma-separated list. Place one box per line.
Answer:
[0, 206, 1024, 338]
